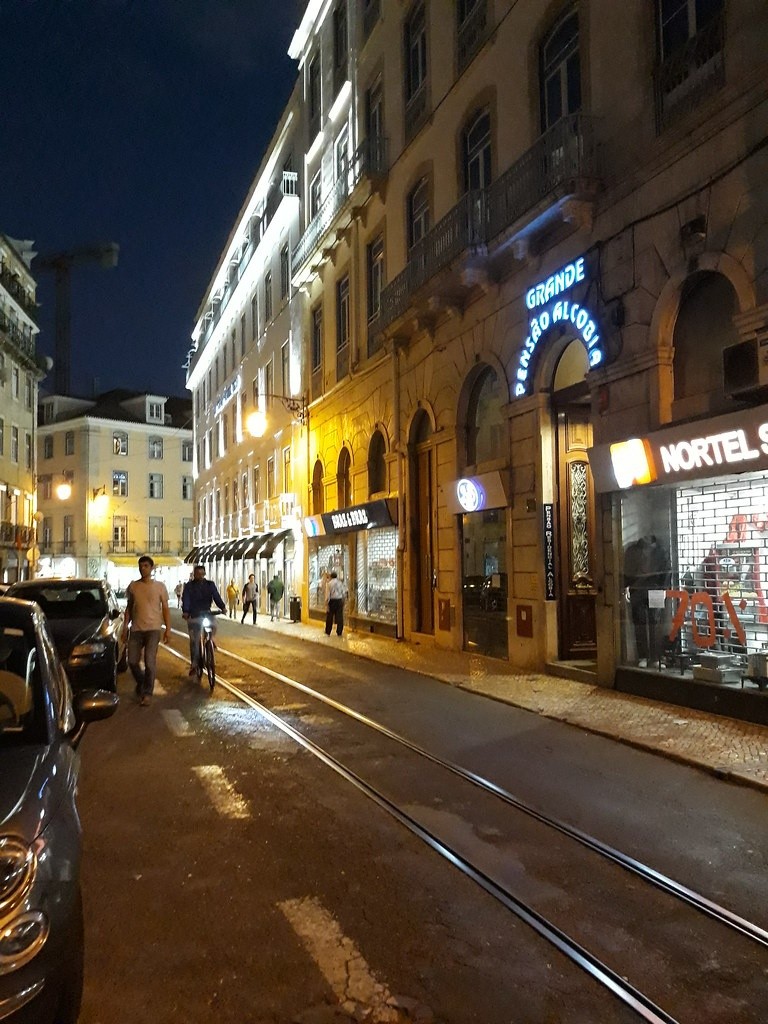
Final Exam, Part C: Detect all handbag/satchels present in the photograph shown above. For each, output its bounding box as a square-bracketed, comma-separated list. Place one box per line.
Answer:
[116, 643, 128, 673]
[177, 594, 180, 597]
[243, 603, 249, 613]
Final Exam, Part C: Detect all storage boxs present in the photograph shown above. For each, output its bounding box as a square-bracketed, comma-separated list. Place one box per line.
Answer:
[697, 651, 734, 669]
[747, 651, 768, 678]
[692, 665, 744, 684]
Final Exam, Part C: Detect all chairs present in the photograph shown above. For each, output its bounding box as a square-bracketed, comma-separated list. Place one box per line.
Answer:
[27, 594, 47, 601]
[75, 592, 96, 601]
[658, 625, 695, 676]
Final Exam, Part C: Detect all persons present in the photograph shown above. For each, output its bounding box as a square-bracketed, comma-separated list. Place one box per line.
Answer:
[173, 579, 182, 609]
[624, 535, 670, 669]
[241, 573, 259, 625]
[226, 577, 240, 619]
[266, 573, 285, 621]
[181, 565, 227, 678]
[121, 556, 171, 707]
[324, 571, 344, 637]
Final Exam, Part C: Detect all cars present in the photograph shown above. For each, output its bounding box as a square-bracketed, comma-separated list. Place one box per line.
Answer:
[2, 577, 130, 694]
[0, 594, 120, 1024]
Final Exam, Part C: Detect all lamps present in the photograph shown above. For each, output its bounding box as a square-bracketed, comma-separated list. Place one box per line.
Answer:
[244, 393, 305, 437]
[57, 469, 74, 500]
[93, 484, 109, 513]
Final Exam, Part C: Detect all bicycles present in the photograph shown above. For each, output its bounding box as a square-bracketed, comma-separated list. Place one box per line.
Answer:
[186, 612, 224, 690]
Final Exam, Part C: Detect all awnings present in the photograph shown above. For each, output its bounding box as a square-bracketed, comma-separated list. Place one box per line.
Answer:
[183, 528, 292, 564]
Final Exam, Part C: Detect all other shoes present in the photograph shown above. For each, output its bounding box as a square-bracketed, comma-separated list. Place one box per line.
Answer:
[135, 682, 143, 695]
[141, 693, 151, 706]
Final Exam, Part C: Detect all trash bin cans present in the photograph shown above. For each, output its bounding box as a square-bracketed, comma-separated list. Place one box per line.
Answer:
[289, 596, 301, 622]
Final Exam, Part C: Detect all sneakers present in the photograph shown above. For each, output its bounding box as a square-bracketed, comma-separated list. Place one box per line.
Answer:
[639, 658, 647, 667]
[654, 660, 667, 669]
